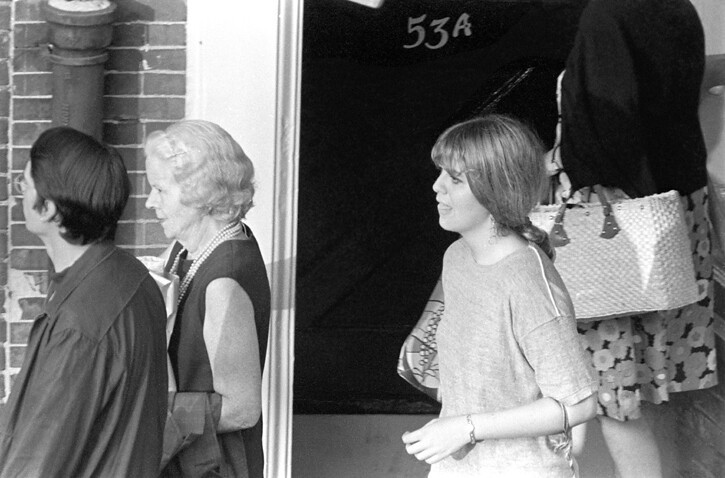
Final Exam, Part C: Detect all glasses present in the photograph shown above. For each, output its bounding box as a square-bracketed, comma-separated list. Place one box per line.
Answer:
[14, 173, 38, 195]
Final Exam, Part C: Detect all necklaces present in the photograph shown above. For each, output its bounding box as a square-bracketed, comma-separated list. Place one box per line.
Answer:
[168, 220, 243, 307]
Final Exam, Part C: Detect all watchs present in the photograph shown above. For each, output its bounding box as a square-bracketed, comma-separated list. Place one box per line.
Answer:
[550, 142, 564, 172]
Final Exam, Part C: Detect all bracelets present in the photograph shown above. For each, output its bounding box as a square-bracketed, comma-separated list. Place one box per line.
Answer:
[465, 413, 477, 445]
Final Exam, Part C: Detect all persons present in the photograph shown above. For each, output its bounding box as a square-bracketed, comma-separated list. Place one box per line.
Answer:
[143, 121, 271, 478]
[400, 112, 599, 478]
[0, 127, 167, 478]
[540, 0, 717, 478]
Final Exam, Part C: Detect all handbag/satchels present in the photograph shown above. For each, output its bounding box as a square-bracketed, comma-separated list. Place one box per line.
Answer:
[396, 272, 445, 402]
[527, 182, 701, 323]
